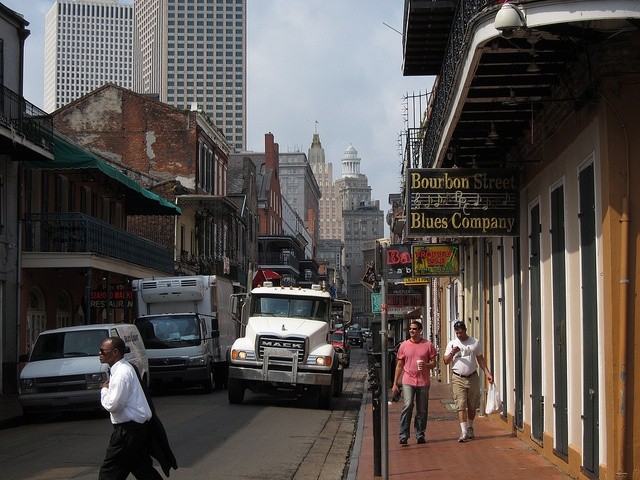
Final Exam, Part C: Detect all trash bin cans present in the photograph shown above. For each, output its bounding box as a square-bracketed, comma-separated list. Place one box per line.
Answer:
[372, 353, 392, 393]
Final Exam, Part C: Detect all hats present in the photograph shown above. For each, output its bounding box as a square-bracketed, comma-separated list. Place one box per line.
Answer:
[454, 321, 466, 328]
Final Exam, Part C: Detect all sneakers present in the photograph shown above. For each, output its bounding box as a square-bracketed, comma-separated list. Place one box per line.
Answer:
[416, 437, 425, 443]
[398, 436, 408, 444]
[459, 433, 468, 443]
[467, 429, 474, 439]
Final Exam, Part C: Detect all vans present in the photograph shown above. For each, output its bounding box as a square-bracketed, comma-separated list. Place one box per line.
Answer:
[17, 322, 149, 421]
[363, 329, 370, 338]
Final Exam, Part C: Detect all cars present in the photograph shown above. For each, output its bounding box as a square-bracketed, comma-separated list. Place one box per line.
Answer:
[363, 334, 366, 343]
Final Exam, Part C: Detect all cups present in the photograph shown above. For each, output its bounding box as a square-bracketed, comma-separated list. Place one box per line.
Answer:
[417, 360, 424, 371]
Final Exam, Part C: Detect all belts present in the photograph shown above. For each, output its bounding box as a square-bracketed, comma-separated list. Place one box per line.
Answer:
[452, 371, 477, 379]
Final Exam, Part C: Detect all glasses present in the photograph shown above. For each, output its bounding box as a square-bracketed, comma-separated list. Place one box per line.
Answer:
[98, 348, 111, 355]
[408, 327, 418, 330]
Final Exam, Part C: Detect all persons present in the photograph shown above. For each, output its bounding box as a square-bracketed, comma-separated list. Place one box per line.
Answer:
[443, 321, 494, 442]
[98, 337, 178, 480]
[392, 321, 438, 445]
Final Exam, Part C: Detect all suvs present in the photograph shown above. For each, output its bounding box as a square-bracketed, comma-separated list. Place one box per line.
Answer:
[346, 330, 363, 348]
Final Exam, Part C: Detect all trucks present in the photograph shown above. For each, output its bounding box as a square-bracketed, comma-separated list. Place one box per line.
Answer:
[129, 275, 240, 396]
[226, 277, 354, 409]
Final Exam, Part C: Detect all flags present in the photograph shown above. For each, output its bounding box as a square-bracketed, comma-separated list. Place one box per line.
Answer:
[263, 269, 282, 280]
[252, 266, 267, 285]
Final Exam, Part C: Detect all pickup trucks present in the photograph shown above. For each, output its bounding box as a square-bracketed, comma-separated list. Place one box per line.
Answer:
[328, 329, 352, 369]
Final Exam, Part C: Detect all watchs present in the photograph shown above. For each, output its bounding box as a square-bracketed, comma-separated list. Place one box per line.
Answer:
[450, 352, 454, 356]
